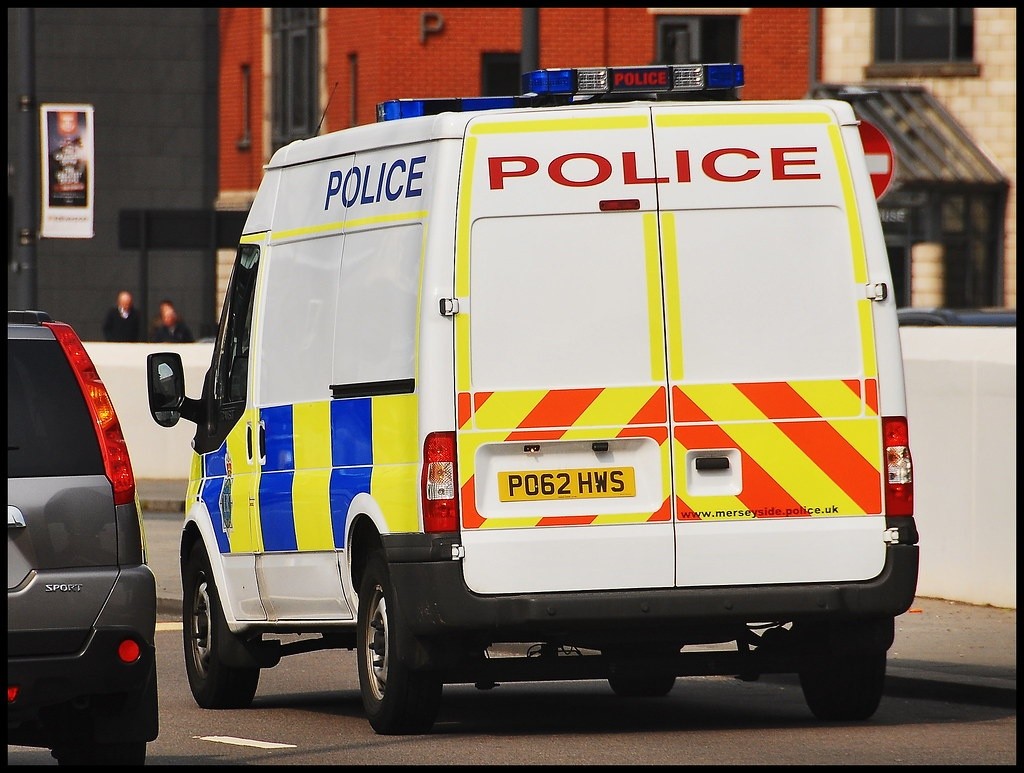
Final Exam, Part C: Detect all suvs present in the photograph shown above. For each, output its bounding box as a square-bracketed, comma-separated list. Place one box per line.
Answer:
[5, 308, 160, 772]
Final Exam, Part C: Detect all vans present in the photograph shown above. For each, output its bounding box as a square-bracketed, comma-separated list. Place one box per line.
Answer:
[140, 63, 925, 740]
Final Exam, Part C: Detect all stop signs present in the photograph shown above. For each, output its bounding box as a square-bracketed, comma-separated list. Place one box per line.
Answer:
[854, 116, 896, 203]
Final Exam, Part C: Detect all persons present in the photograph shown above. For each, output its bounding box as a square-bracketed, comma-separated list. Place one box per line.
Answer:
[149, 300, 194, 344]
[103, 291, 143, 342]
